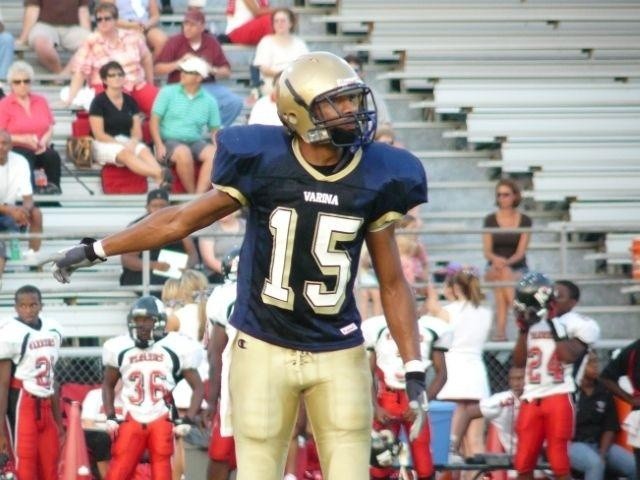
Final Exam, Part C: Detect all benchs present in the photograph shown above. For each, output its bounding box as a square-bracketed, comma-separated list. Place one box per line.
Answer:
[404, 464, 585, 480]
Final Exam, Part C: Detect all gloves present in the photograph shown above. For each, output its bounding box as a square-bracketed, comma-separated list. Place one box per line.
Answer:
[400, 359, 432, 442]
[169, 415, 196, 438]
[33, 239, 111, 287]
[103, 417, 121, 443]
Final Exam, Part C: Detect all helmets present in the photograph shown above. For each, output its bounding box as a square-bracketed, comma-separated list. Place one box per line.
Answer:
[126, 294, 169, 339]
[274, 49, 368, 144]
[513, 271, 555, 316]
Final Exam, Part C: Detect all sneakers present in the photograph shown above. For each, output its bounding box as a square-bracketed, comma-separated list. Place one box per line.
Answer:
[159, 166, 177, 185]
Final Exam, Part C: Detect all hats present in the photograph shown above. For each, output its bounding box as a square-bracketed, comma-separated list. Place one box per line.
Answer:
[177, 55, 210, 78]
[142, 189, 170, 209]
[182, 10, 207, 25]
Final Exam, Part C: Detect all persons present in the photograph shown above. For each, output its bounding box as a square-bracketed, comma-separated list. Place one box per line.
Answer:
[0, 0, 453, 480]
[439, 182, 640, 480]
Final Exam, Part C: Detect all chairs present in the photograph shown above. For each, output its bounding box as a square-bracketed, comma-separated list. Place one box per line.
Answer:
[58, 381, 161, 480]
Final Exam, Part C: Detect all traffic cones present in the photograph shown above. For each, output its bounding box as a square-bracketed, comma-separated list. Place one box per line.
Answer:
[52, 397, 97, 480]
[480, 422, 508, 480]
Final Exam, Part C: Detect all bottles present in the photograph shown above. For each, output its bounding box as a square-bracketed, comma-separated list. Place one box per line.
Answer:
[12, 238, 20, 259]
[37, 169, 47, 187]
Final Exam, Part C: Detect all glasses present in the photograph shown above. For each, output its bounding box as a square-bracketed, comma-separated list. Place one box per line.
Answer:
[95, 14, 113, 22]
[12, 78, 31, 84]
[496, 191, 514, 198]
[106, 72, 125, 77]
